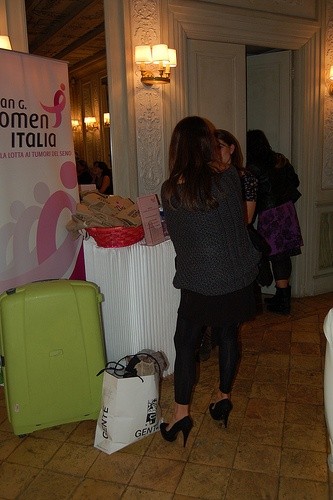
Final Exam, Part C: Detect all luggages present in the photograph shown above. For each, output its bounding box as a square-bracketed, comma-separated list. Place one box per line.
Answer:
[0, 278, 107, 438]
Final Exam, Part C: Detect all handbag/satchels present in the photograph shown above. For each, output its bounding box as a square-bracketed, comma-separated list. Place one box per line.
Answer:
[93, 353, 163, 455]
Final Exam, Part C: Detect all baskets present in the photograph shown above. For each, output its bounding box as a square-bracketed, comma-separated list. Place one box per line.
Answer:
[84, 225, 144, 248]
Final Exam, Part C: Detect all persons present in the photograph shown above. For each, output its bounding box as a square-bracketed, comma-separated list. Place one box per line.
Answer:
[77, 160, 113, 195]
[246, 128, 302, 317]
[217, 130, 257, 224]
[160, 115, 257, 449]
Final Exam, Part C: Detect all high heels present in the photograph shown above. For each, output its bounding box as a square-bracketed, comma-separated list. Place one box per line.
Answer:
[209, 399, 233, 428]
[160, 415, 193, 447]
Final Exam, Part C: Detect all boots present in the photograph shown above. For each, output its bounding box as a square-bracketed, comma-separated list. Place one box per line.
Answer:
[264, 284, 292, 315]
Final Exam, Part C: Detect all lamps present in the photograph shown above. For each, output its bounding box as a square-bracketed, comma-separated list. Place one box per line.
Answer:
[71, 120, 79, 130]
[135, 44, 177, 84]
[84, 117, 98, 130]
[104, 113, 110, 128]
